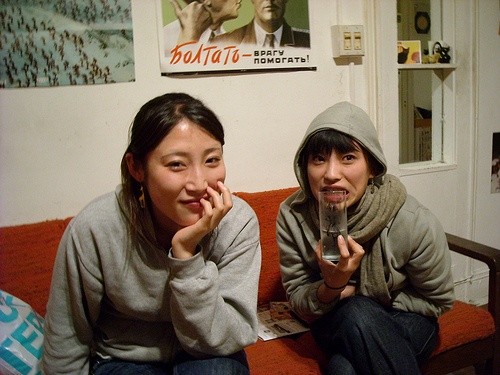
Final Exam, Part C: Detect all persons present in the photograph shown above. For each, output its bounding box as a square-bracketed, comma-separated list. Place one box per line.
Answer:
[162, 0, 243, 58]
[276, 102, 456, 375]
[208, 0, 310, 50]
[39, 92, 263, 375]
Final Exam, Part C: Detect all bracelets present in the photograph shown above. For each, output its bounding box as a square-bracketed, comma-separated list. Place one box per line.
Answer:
[323, 280, 346, 291]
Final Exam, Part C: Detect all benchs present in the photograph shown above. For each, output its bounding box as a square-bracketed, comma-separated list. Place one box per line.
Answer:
[0, 186, 500, 375]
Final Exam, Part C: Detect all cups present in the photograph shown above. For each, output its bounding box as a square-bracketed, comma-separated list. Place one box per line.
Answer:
[318, 191, 348, 261]
